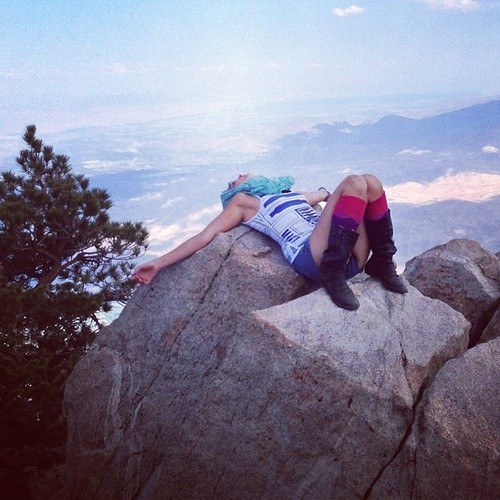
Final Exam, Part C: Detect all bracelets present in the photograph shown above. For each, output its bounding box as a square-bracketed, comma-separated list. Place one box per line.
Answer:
[317, 187, 330, 202]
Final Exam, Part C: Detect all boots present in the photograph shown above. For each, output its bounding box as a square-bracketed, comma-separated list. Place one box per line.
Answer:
[319, 223, 361, 310]
[364, 208, 409, 294]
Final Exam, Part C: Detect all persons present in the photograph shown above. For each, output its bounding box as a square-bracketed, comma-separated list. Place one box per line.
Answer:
[131, 173, 408, 310]
